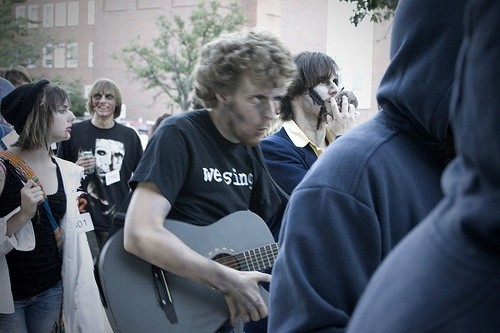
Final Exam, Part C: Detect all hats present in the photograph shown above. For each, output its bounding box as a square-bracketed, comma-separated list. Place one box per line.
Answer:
[0, 79, 50, 135]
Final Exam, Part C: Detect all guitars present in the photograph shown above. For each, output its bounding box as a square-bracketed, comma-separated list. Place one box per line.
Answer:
[91, 208, 278, 333]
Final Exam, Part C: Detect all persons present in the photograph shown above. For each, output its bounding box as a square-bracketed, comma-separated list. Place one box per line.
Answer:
[257, 51, 360, 243]
[56, 78, 143, 262]
[124, 27, 298, 333]
[4, 62, 32, 87]
[0, 79, 113, 333]
[267, 0, 500, 333]
